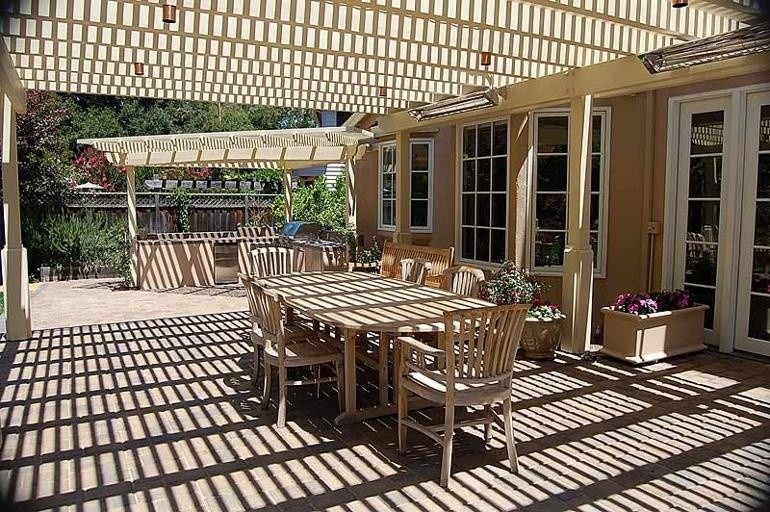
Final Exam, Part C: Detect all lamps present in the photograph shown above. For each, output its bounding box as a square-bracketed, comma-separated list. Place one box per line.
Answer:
[407, 72, 507, 121]
[637, 20, 770, 75]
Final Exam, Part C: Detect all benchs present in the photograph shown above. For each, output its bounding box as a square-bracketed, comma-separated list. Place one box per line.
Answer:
[378, 239, 454, 290]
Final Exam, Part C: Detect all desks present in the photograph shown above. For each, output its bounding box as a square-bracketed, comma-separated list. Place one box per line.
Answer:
[250, 270, 499, 428]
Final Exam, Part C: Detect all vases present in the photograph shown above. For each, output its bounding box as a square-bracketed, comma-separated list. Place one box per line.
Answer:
[519, 315, 566, 359]
[600, 303, 710, 365]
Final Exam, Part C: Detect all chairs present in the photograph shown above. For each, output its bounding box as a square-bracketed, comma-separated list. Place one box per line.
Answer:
[250, 248, 307, 317]
[399, 256, 433, 286]
[237, 270, 321, 402]
[395, 303, 532, 490]
[419, 265, 486, 366]
[251, 280, 345, 429]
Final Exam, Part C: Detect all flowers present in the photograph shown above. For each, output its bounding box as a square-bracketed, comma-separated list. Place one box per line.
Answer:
[614, 288, 694, 315]
[521, 293, 567, 319]
